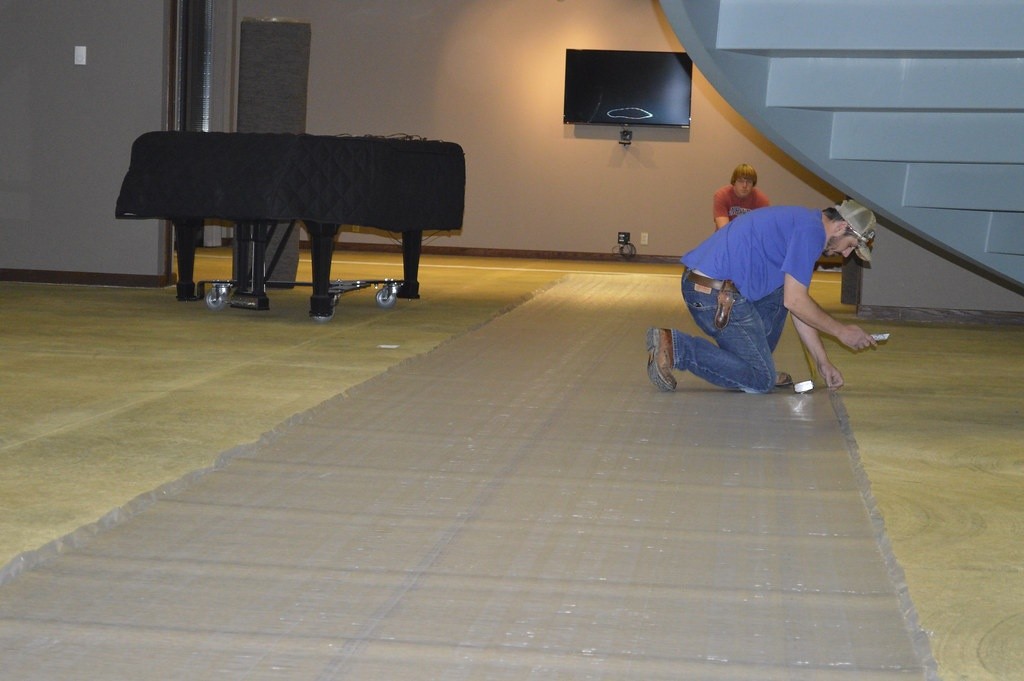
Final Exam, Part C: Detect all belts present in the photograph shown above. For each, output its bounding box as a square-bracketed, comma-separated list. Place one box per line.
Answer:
[684, 268, 724, 291]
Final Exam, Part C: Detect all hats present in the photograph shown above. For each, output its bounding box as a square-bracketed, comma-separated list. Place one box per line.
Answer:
[833, 199, 876, 261]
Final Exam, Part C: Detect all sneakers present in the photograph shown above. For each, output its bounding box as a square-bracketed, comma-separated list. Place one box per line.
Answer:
[646, 327, 678, 392]
[774, 370, 793, 385]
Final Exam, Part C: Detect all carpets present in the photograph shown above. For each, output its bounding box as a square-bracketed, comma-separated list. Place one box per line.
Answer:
[0, 270, 945, 681]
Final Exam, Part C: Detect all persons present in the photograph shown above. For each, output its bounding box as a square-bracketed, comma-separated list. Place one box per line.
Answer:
[710, 163, 770, 234]
[646, 199, 878, 395]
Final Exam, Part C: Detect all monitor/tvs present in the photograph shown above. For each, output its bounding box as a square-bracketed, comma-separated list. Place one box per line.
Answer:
[563, 48, 692, 128]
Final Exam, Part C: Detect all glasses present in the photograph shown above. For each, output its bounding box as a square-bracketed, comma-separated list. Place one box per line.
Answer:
[735, 178, 754, 184]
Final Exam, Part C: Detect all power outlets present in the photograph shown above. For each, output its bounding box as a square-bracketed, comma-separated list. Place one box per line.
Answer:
[618, 232, 630, 245]
[641, 232, 649, 245]
